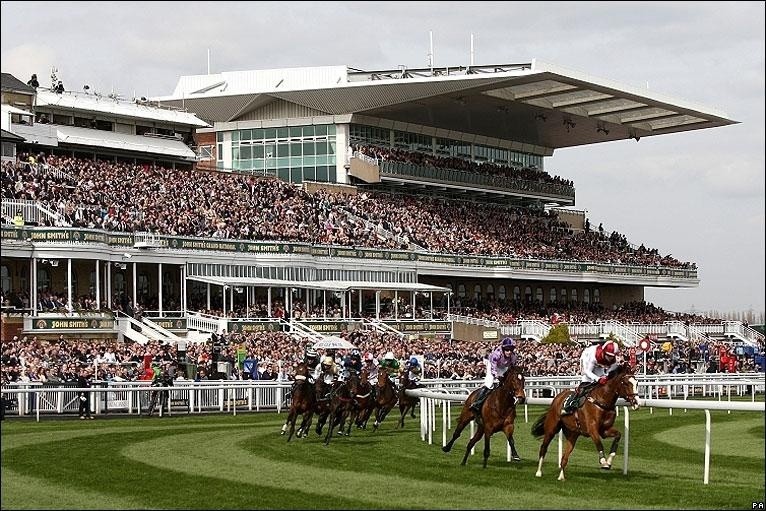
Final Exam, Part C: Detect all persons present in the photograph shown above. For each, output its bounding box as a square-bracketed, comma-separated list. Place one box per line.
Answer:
[0, 73, 764, 420]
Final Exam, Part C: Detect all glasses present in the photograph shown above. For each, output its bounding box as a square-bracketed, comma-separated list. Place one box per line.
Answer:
[504, 347, 514, 351]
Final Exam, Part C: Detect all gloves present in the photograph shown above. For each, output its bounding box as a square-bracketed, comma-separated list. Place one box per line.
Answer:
[599, 378, 607, 385]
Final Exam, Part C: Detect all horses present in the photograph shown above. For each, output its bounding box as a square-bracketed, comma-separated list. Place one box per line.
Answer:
[441, 362, 526, 469]
[531, 364, 641, 480]
[280, 360, 425, 446]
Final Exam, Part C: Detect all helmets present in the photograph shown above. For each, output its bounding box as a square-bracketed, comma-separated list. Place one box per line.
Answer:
[306, 350, 418, 366]
[502, 337, 515, 347]
[602, 341, 618, 356]
